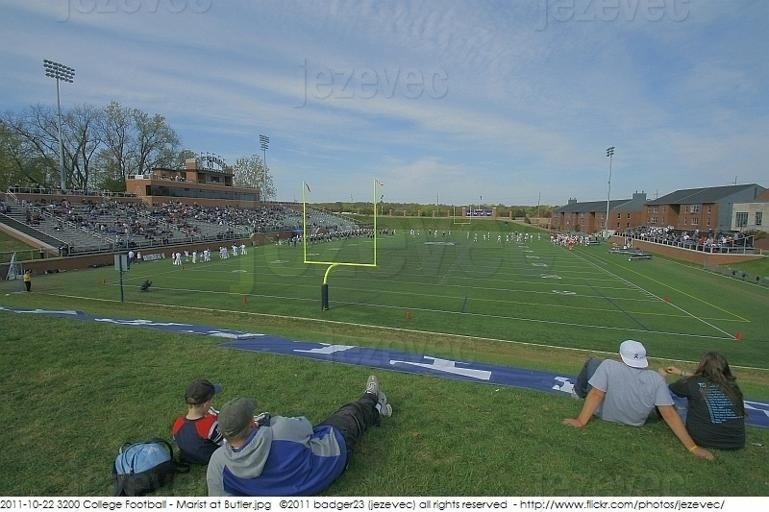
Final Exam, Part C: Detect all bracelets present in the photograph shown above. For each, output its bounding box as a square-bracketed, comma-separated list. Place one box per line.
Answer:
[679, 367, 683, 377]
[687, 446, 699, 454]
[683, 369, 688, 379]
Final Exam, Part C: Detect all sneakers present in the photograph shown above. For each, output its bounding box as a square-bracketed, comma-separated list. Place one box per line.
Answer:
[375, 391, 392, 418]
[253, 411, 272, 422]
[571, 386, 583, 401]
[208, 406, 219, 415]
[365, 375, 379, 402]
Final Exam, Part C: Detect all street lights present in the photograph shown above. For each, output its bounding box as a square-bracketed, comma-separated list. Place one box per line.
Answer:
[603, 146, 615, 231]
[259, 135, 270, 201]
[42, 58, 76, 194]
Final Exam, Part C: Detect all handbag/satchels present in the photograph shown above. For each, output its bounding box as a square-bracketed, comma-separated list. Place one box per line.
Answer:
[113, 438, 174, 496]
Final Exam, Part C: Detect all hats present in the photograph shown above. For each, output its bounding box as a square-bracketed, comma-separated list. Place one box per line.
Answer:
[185, 379, 221, 404]
[620, 340, 648, 369]
[218, 397, 257, 435]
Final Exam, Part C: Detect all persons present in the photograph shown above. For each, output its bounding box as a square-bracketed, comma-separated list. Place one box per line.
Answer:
[170, 377, 274, 468]
[614, 224, 753, 253]
[21, 269, 35, 293]
[202, 375, 395, 496]
[139, 279, 153, 291]
[408, 226, 612, 253]
[560, 337, 717, 463]
[656, 350, 748, 453]
[0, 195, 399, 281]
